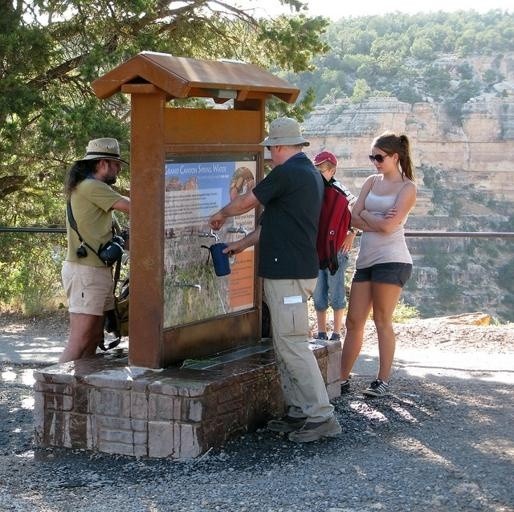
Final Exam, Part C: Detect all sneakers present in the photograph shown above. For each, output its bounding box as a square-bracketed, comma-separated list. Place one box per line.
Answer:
[342, 380, 349, 391]
[289, 415, 343, 442]
[315, 332, 341, 340]
[363, 379, 391, 396]
[268, 414, 307, 433]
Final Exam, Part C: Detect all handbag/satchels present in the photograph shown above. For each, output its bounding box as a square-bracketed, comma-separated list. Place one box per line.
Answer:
[107, 287, 130, 337]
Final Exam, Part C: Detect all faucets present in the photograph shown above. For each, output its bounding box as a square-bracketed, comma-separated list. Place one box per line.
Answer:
[226, 222, 249, 238]
[196, 227, 222, 242]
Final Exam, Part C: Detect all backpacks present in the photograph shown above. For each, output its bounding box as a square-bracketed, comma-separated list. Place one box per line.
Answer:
[318, 173, 352, 274]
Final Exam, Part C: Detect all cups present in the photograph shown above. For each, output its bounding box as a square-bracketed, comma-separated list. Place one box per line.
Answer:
[210, 243, 230, 275]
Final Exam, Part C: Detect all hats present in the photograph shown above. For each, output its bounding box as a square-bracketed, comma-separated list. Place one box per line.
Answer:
[315, 151, 337, 166]
[74, 137, 130, 167]
[259, 118, 311, 146]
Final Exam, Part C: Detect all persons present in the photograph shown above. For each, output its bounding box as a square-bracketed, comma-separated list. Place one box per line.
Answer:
[341, 135, 418, 397]
[209, 117, 341, 442]
[313, 149, 358, 343]
[57, 136, 131, 364]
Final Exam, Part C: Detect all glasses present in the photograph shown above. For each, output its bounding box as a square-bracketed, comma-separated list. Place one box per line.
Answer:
[369, 153, 389, 162]
